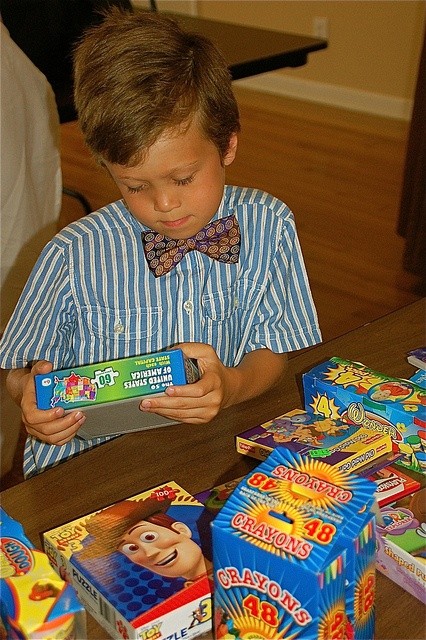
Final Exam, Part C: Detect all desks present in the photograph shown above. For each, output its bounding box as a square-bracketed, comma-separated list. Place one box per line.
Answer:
[0, 296, 425, 640]
[2, 2, 328, 218]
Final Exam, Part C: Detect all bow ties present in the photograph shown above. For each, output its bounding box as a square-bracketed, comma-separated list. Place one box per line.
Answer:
[140, 214, 242, 279]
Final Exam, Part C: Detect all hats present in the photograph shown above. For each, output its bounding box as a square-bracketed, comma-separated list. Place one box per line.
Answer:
[79, 490, 172, 562]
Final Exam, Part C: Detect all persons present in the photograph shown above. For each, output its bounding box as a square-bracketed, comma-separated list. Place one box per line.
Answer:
[0, 4, 323, 480]
